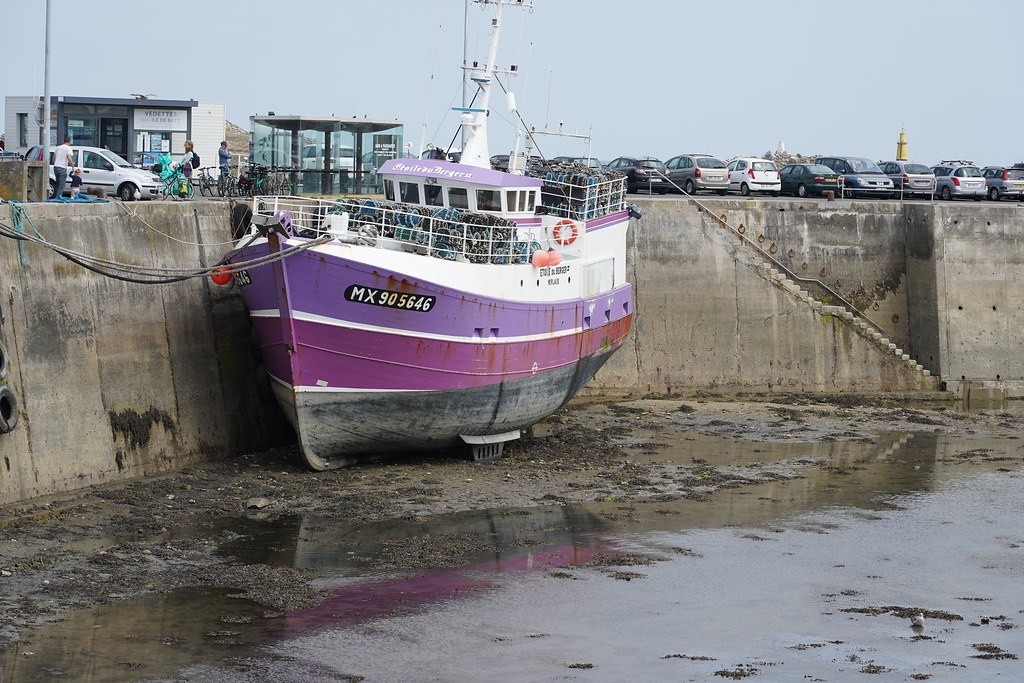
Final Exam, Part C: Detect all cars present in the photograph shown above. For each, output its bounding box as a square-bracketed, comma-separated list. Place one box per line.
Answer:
[727, 158, 781, 197]
[362, 150, 419, 188]
[779, 163, 845, 199]
[417, 148, 628, 197]
[24, 145, 163, 201]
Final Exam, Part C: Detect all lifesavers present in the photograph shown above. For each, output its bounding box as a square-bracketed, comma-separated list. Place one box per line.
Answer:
[757, 235, 766, 244]
[787, 249, 796, 258]
[737, 225, 746, 234]
[769, 244, 779, 253]
[553, 219, 579, 247]
[801, 263, 808, 270]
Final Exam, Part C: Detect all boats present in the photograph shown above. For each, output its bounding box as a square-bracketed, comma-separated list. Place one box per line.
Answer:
[211, 0, 631, 472]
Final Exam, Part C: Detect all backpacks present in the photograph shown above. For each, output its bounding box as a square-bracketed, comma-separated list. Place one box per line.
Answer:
[186, 150, 200, 169]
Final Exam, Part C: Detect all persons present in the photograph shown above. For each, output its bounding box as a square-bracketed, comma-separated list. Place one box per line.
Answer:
[172, 140, 195, 200]
[53, 136, 83, 200]
[218, 140, 232, 190]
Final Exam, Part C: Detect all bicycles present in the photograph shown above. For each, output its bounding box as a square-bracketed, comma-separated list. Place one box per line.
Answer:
[151, 162, 299, 200]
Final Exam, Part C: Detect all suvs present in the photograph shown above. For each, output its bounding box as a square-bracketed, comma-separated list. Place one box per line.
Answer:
[930, 160, 988, 202]
[298, 143, 365, 188]
[877, 161, 937, 200]
[663, 154, 732, 196]
[604, 155, 670, 195]
[981, 166, 1024, 202]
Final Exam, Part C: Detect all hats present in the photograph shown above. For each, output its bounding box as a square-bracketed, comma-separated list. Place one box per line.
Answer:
[222, 141, 228, 146]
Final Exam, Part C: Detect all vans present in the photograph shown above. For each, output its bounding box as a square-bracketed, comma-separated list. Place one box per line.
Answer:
[814, 157, 894, 200]
